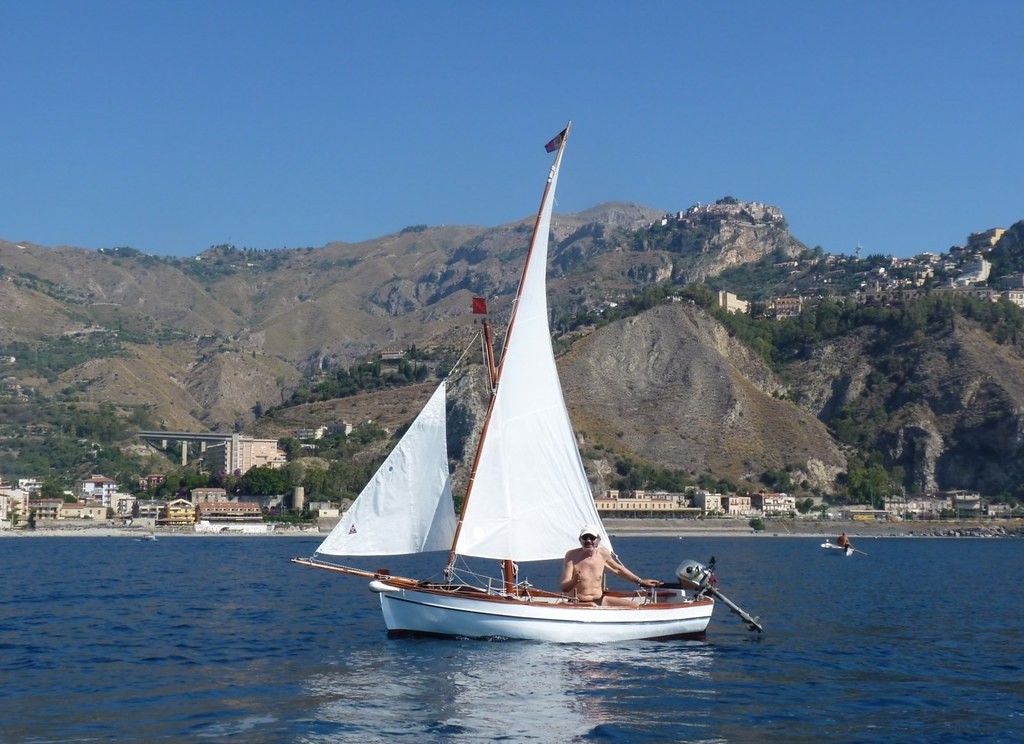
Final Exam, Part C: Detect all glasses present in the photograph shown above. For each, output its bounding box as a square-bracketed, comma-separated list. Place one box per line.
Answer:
[580, 534, 599, 540]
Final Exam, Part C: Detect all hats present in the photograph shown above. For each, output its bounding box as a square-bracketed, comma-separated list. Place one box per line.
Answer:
[580, 525, 601, 540]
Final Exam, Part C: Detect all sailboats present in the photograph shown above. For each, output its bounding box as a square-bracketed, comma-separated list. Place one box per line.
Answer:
[287, 112, 763, 644]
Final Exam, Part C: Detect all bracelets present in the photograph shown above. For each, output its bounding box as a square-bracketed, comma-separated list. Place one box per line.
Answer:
[637, 577, 641, 586]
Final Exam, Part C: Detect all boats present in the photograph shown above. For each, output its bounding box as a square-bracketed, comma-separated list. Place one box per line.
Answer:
[820, 539, 855, 558]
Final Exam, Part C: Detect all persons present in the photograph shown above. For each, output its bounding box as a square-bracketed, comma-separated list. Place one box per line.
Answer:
[561, 524, 659, 607]
[837, 532, 849, 547]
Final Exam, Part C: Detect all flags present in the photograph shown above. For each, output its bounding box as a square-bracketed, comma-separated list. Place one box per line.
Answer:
[545, 127, 567, 153]
